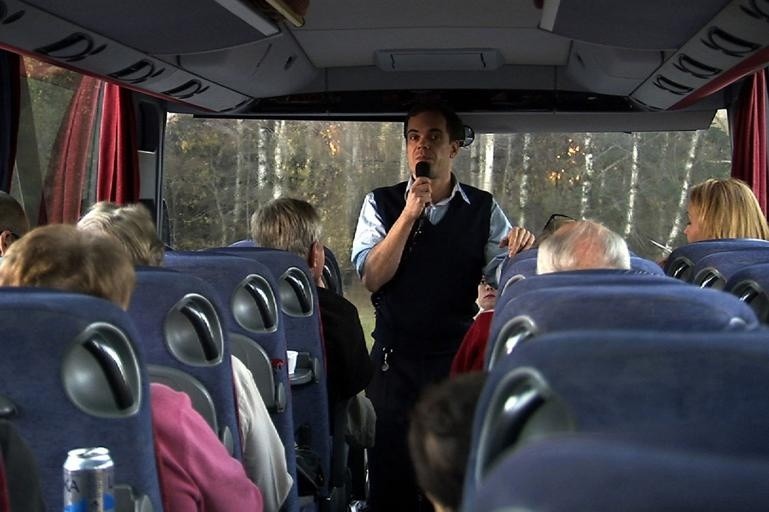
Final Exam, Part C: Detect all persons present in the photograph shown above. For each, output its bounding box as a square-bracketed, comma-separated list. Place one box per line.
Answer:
[0, 223, 263, 512]
[453, 220, 631, 372]
[250, 201, 376, 511]
[350, 98, 538, 511]
[473, 272, 498, 312]
[0, 191, 28, 256]
[682, 179, 769, 243]
[403, 368, 508, 511]
[78, 200, 293, 512]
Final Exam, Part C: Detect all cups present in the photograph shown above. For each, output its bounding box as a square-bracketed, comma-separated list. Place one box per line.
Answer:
[287, 351, 298, 373]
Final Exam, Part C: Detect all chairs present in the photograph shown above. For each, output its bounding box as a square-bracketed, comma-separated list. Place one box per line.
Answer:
[466, 435, 769, 512]
[201, 249, 336, 512]
[230, 237, 342, 294]
[464, 236, 769, 490]
[0, 287, 164, 511]
[160, 252, 301, 510]
[125, 270, 246, 476]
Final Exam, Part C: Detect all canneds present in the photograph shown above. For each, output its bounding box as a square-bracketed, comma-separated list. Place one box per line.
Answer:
[62, 446, 118, 512]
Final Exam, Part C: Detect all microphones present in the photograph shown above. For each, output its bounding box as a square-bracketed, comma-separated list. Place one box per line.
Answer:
[415, 161, 431, 208]
